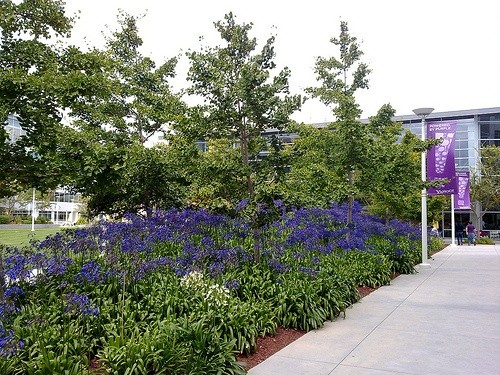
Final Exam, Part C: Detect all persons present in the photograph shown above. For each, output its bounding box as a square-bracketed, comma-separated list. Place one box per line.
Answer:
[466, 221, 476, 246]
[456, 221, 465, 245]
[432, 226, 438, 237]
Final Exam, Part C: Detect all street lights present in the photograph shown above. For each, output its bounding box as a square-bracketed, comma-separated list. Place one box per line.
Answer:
[412, 107, 435, 267]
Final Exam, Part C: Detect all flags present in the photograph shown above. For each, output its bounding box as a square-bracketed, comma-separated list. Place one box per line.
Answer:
[425, 121, 459, 196]
[454, 170, 471, 210]
